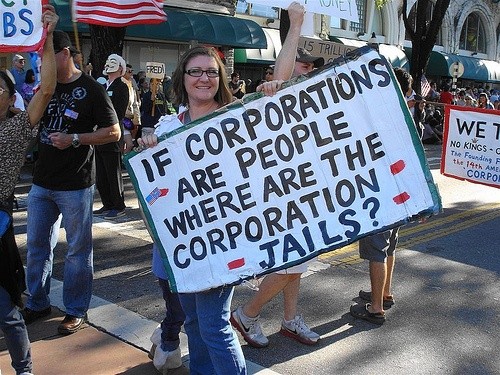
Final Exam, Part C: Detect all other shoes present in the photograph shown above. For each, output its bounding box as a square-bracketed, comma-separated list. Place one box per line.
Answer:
[103, 209, 126, 219]
[93, 205, 112, 216]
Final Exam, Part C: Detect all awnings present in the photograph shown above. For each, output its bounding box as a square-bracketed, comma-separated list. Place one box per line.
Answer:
[234, 26, 283, 64]
[401, 45, 500, 83]
[48, 0, 268, 50]
[329, 35, 410, 72]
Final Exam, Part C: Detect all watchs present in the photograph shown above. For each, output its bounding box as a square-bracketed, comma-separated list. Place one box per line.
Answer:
[73, 134, 80, 148]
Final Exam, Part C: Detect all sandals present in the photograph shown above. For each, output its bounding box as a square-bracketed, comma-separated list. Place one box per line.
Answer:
[359, 289, 394, 306]
[350, 303, 386, 325]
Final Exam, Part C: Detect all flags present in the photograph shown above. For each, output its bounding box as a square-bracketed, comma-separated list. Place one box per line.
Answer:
[71, 0, 168, 28]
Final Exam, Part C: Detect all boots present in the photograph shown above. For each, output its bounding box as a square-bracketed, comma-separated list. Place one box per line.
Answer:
[148, 327, 190, 375]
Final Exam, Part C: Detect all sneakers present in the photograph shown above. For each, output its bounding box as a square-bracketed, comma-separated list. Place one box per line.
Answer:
[279, 314, 320, 345]
[229, 306, 269, 348]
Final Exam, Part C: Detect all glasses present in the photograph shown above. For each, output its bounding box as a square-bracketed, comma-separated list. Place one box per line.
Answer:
[465, 99, 470, 100]
[0, 88, 13, 96]
[183, 69, 220, 78]
[16, 61, 25, 64]
[460, 95, 464, 97]
[264, 71, 273, 75]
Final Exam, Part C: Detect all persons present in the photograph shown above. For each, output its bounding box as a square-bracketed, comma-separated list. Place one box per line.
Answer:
[349, 66, 413, 328]
[137, 45, 286, 375]
[10, 30, 121, 336]
[0, 1, 500, 375]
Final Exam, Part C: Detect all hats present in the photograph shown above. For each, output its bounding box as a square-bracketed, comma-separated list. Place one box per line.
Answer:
[52, 30, 80, 54]
[13, 54, 26, 60]
[295, 47, 324, 68]
[264, 64, 275, 71]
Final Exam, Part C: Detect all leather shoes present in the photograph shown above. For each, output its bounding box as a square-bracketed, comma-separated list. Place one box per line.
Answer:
[19, 307, 51, 325]
[58, 313, 86, 334]
